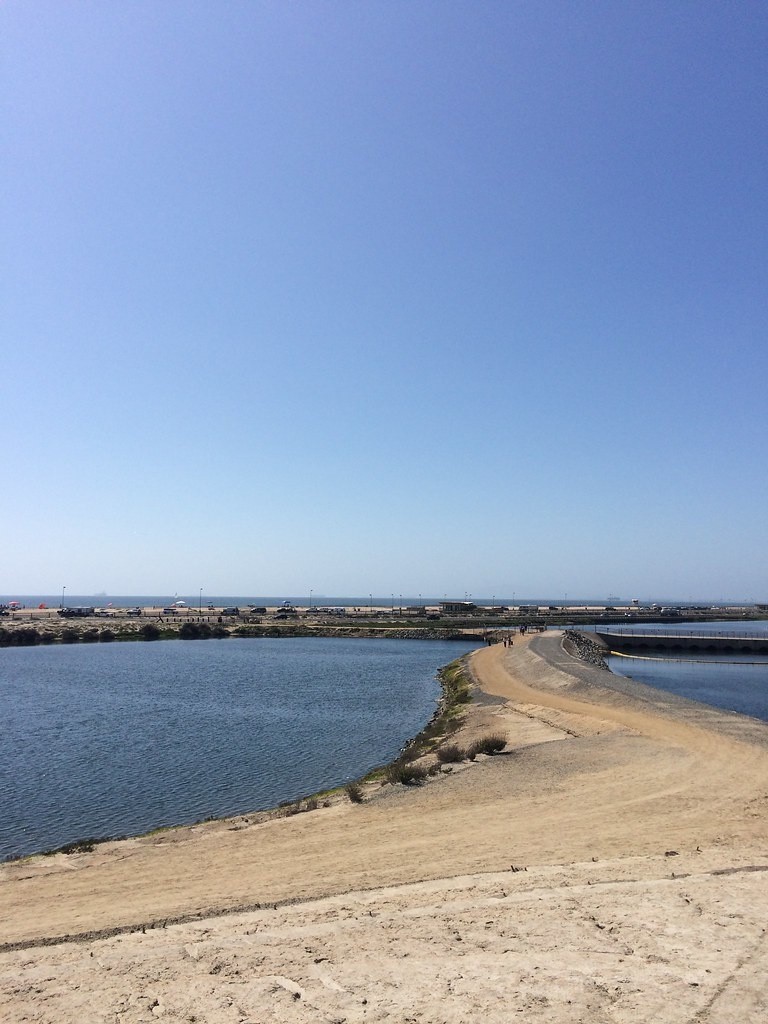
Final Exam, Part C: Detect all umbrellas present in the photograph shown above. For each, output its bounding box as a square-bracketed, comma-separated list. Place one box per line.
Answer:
[9, 601, 20, 605]
[206, 600, 212, 606]
[176, 600, 185, 607]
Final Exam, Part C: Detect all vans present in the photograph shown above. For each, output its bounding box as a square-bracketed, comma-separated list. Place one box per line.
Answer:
[164, 608, 179, 614]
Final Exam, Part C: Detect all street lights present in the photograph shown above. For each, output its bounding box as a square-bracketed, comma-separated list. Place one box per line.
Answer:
[200, 587, 203, 610]
[62, 586, 66, 608]
[391, 592, 473, 606]
[310, 589, 313, 607]
[370, 593, 372, 612]
[492, 595, 495, 608]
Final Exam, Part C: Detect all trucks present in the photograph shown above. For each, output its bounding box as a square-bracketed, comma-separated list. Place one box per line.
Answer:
[223, 607, 238, 616]
[64, 607, 91, 617]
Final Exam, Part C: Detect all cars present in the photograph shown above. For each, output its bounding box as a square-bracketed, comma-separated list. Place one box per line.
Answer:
[277, 607, 346, 615]
[600, 612, 610, 617]
[625, 612, 638, 617]
[640, 605, 709, 617]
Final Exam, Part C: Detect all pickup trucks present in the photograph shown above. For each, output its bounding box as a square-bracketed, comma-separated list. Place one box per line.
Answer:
[93, 611, 114, 618]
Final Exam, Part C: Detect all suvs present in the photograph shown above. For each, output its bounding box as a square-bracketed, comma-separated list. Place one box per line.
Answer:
[126, 608, 142, 615]
[250, 607, 267, 613]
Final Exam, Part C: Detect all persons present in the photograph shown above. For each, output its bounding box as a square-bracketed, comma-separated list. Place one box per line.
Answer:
[520, 626, 524, 636]
[138, 608, 141, 615]
[503, 637, 511, 647]
